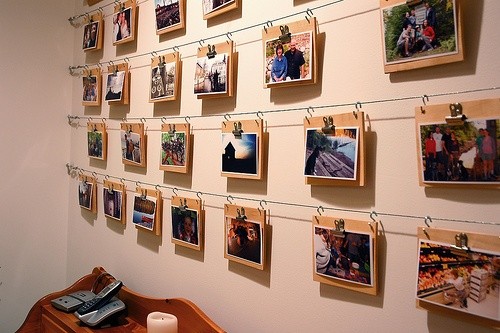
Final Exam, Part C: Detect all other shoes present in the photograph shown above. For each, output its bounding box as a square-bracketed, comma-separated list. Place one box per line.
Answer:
[427, 47, 434, 51]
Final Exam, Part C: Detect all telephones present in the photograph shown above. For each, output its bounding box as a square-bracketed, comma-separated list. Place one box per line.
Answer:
[74, 280, 127, 329]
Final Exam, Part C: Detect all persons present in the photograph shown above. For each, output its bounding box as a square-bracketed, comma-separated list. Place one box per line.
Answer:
[442, 270, 470, 309]
[315, 228, 365, 282]
[227, 225, 249, 261]
[82, 11, 306, 165]
[423, 127, 498, 182]
[175, 215, 198, 245]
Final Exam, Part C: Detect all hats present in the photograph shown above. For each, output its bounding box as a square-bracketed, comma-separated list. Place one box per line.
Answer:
[415, 25, 419, 28]
